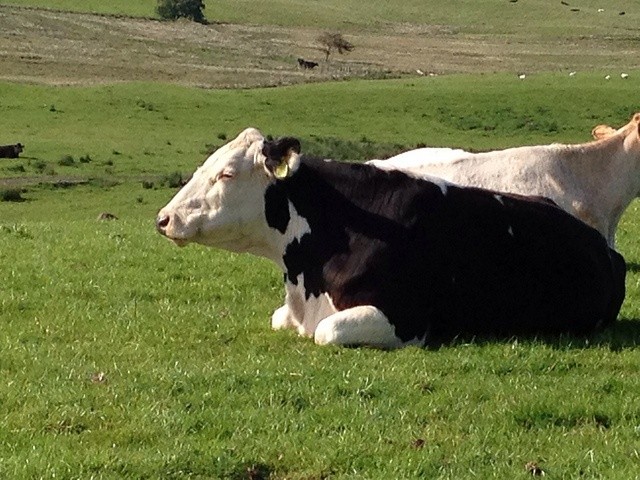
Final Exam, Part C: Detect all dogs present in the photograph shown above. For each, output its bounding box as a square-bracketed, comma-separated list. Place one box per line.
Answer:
[297, 57, 318, 71]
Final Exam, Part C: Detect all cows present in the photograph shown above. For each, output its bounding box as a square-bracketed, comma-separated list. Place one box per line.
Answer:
[1, 142, 24, 161]
[155, 128, 628, 352]
[365, 113, 640, 250]
